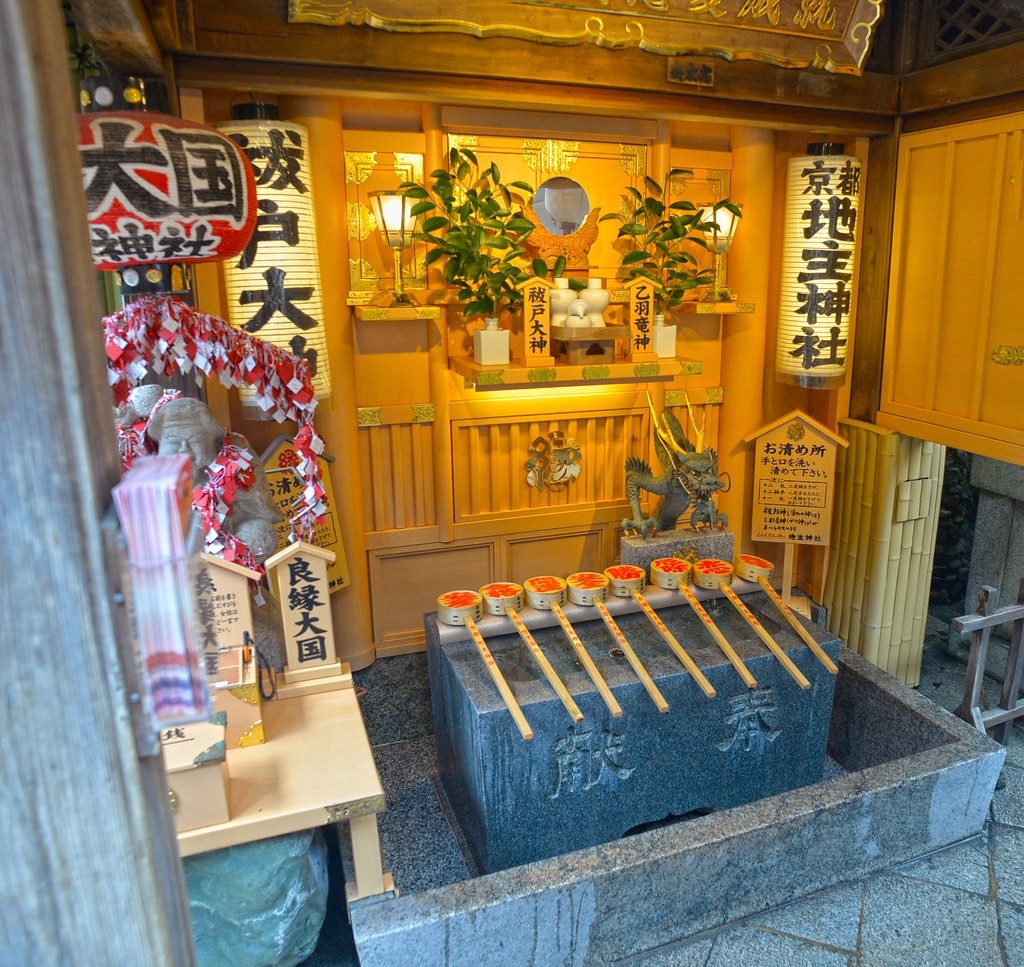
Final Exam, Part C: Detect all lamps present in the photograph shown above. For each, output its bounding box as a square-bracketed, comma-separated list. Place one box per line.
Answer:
[370, 191, 420, 304]
[697, 202, 738, 301]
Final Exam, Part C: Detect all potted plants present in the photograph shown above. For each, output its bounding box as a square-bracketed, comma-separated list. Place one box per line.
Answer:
[599, 169, 741, 360]
[400, 142, 536, 367]
